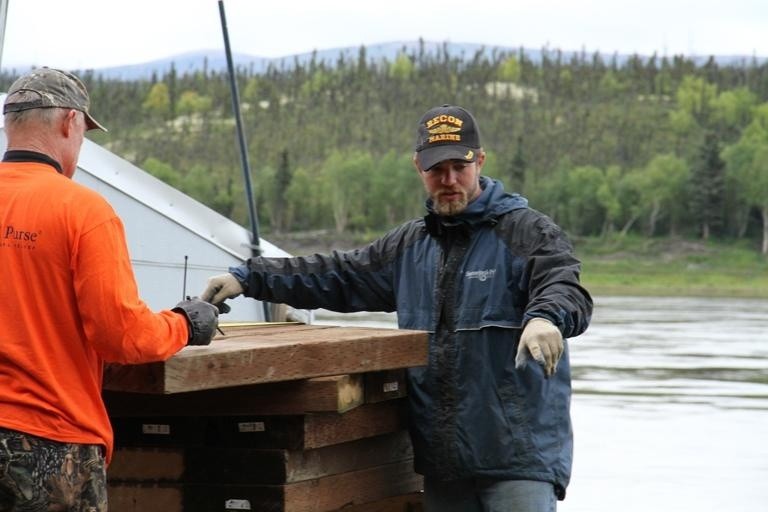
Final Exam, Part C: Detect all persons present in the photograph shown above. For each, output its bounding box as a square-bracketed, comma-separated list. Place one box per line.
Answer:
[0, 66, 218, 512]
[200, 103, 594, 512]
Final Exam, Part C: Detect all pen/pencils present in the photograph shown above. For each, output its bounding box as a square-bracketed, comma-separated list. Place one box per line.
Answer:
[185, 295, 226, 337]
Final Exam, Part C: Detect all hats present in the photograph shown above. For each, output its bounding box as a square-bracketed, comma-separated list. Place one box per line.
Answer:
[3, 66, 108, 132]
[416, 104, 482, 172]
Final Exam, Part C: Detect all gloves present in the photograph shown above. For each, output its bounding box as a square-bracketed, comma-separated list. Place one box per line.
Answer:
[171, 296, 218, 346]
[200, 272, 245, 314]
[514, 317, 564, 379]
